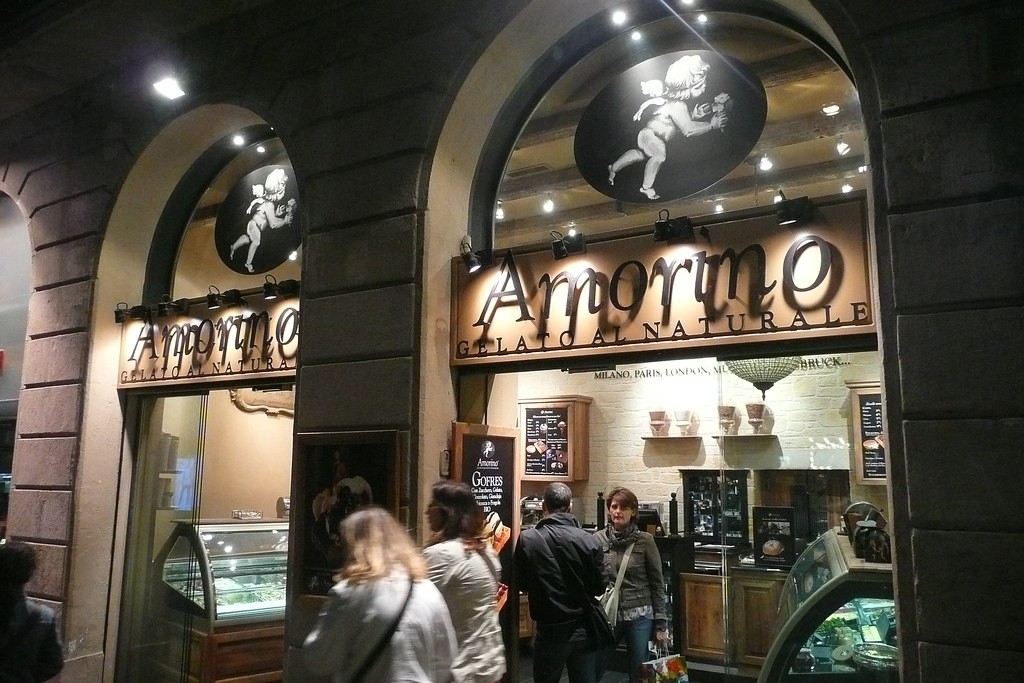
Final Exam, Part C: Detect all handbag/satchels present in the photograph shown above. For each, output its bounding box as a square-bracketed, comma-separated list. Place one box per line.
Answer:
[595, 588, 619, 629]
[583, 598, 616, 648]
[643, 643, 688, 683]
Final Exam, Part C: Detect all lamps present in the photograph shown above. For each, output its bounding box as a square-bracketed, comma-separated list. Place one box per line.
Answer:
[550, 231, 585, 261]
[115, 302, 151, 323]
[264, 275, 298, 303]
[726, 356, 801, 401]
[460, 242, 496, 274]
[773, 192, 808, 227]
[158, 294, 191, 316]
[648, 210, 695, 242]
[206, 286, 243, 310]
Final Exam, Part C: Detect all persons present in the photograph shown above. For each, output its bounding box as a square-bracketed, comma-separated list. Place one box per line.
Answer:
[422, 479, 507, 683]
[297, 506, 459, 683]
[592, 485, 670, 683]
[0, 535, 65, 683]
[513, 483, 604, 683]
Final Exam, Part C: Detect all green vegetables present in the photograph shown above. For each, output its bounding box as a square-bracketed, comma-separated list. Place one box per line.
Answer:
[816, 617, 846, 635]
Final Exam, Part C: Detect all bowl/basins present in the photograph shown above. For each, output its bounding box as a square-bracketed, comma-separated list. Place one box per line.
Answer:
[852, 642, 899, 671]
